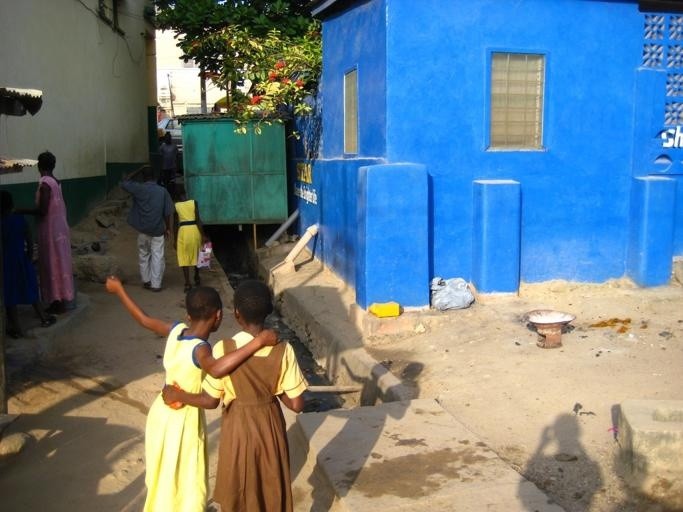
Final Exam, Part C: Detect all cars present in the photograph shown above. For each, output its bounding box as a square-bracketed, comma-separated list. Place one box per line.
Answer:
[156, 116, 183, 151]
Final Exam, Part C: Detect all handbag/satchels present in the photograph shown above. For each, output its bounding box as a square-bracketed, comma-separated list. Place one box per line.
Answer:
[197, 252, 211, 269]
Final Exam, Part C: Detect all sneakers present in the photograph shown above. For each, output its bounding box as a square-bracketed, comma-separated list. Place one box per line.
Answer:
[41, 315, 56, 327]
[184, 283, 192, 293]
[45, 300, 76, 314]
[194, 275, 201, 285]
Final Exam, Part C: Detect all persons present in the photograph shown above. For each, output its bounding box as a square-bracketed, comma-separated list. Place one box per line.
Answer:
[174, 183, 209, 292]
[13, 153, 75, 315]
[120, 164, 175, 290]
[0, 190, 57, 339]
[163, 281, 309, 512]
[157, 132, 179, 186]
[105, 274, 279, 512]
